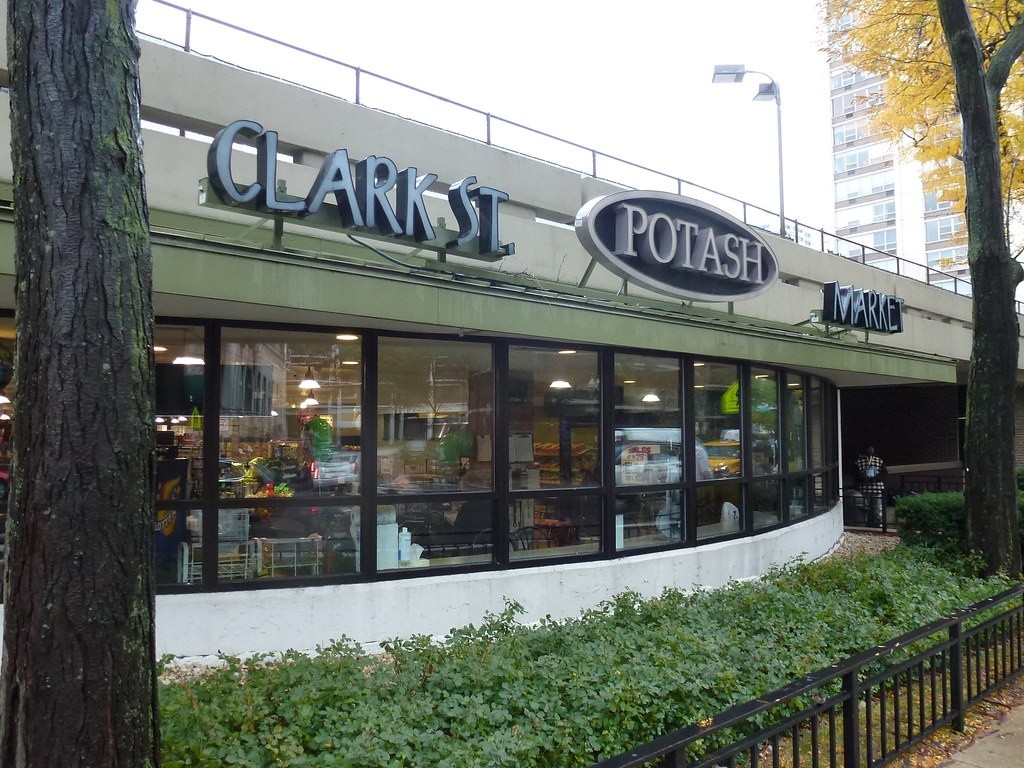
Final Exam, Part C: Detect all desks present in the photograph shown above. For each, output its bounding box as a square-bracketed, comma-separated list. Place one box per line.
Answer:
[622, 521, 681, 537]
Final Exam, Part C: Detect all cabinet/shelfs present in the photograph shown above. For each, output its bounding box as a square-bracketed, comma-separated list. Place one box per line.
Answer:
[178, 537, 323, 583]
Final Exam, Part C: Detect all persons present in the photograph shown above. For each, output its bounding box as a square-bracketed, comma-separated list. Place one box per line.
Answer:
[430, 471, 493, 543]
[852, 444, 885, 528]
[582, 453, 602, 487]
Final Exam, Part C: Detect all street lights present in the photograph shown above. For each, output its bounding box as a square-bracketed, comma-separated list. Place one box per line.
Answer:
[712, 63, 786, 240]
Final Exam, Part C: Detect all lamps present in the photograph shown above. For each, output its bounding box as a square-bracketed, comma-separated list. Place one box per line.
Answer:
[300, 390, 318, 409]
[550, 378, 573, 389]
[641, 391, 662, 403]
[298, 366, 321, 390]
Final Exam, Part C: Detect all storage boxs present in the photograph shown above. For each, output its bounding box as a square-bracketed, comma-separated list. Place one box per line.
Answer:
[191, 508, 250, 541]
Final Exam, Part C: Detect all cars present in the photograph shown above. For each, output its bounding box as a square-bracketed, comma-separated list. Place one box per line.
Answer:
[190, 440, 433, 497]
[608, 424, 753, 508]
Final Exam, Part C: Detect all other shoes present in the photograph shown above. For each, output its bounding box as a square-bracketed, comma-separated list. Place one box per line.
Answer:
[869, 524, 883, 528]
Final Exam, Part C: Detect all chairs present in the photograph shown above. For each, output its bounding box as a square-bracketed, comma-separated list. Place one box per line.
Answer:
[424, 524, 550, 550]
[636, 496, 680, 533]
